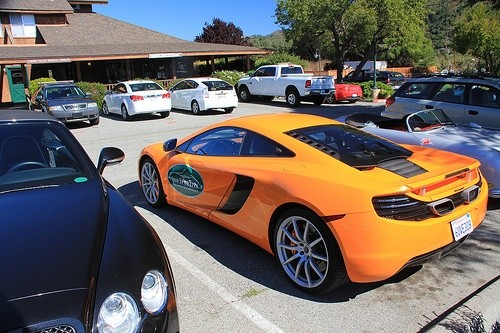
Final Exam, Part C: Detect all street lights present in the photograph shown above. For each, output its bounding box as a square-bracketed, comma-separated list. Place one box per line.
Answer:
[314, 49, 322, 75]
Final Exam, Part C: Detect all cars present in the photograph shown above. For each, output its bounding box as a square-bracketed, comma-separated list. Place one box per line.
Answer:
[29, 81, 100, 126]
[101, 81, 171, 122]
[378, 71, 406, 91]
[168, 77, 238, 115]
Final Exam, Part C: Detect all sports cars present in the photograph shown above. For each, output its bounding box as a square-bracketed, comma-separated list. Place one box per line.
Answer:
[138, 113, 486, 295]
[333, 106, 500, 200]
[324, 82, 362, 104]
[0, 107, 179, 333]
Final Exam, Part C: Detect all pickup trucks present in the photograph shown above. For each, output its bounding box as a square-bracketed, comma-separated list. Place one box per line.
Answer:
[341, 69, 382, 82]
[237, 62, 336, 108]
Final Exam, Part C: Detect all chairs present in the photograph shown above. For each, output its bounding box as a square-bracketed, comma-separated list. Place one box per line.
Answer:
[0, 136, 49, 179]
[250, 135, 280, 155]
[401, 113, 426, 132]
[435, 88, 460, 103]
[61, 89, 71, 97]
[474, 90, 499, 108]
[373, 119, 406, 130]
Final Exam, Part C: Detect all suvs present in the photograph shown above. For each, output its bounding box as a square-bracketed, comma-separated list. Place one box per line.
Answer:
[381, 72, 500, 131]
